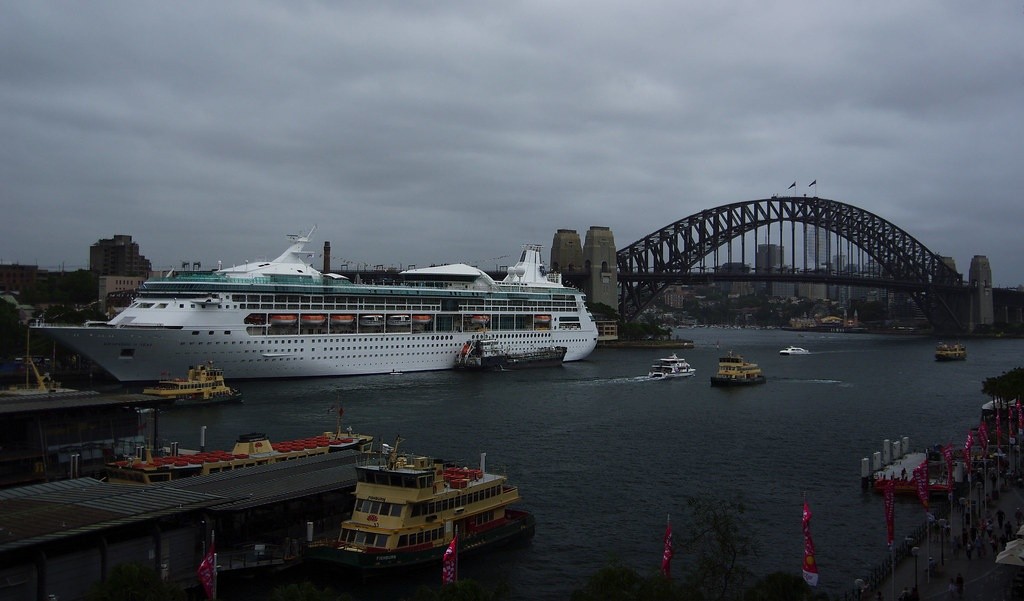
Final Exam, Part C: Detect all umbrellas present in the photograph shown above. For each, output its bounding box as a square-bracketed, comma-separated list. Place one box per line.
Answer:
[995, 538, 1024, 575]
[1016, 523, 1024, 535]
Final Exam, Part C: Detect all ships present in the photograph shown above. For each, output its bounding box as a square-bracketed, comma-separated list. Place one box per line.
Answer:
[28, 243, 599, 388]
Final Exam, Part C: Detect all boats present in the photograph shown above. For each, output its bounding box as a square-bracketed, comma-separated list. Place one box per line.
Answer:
[387, 315, 410, 326]
[360, 315, 383, 327]
[103, 407, 375, 487]
[412, 315, 433, 324]
[301, 315, 325, 326]
[331, 315, 354, 325]
[934, 338, 967, 360]
[648, 353, 695, 380]
[779, 346, 809, 356]
[269, 314, 296, 325]
[710, 348, 767, 385]
[465, 315, 488, 324]
[296, 435, 536, 580]
[534, 315, 549, 323]
[142, 360, 243, 408]
[501, 346, 566, 369]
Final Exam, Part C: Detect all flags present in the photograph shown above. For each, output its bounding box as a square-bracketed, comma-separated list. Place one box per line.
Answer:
[941, 442, 953, 493]
[996, 400, 1024, 448]
[979, 421, 993, 458]
[960, 431, 976, 475]
[788, 181, 795, 189]
[661, 514, 673, 577]
[442, 533, 458, 585]
[802, 500, 819, 587]
[883, 479, 895, 551]
[912, 459, 929, 512]
[809, 179, 816, 187]
[194, 542, 217, 601]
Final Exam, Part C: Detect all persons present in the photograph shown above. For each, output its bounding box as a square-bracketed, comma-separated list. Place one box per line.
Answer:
[862, 586, 920, 601]
[901, 440, 1024, 560]
[948, 572, 964, 599]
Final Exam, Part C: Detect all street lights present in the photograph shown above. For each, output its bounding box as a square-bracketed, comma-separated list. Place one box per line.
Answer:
[938, 482, 983, 566]
[911, 547, 920, 587]
[853, 578, 865, 601]
